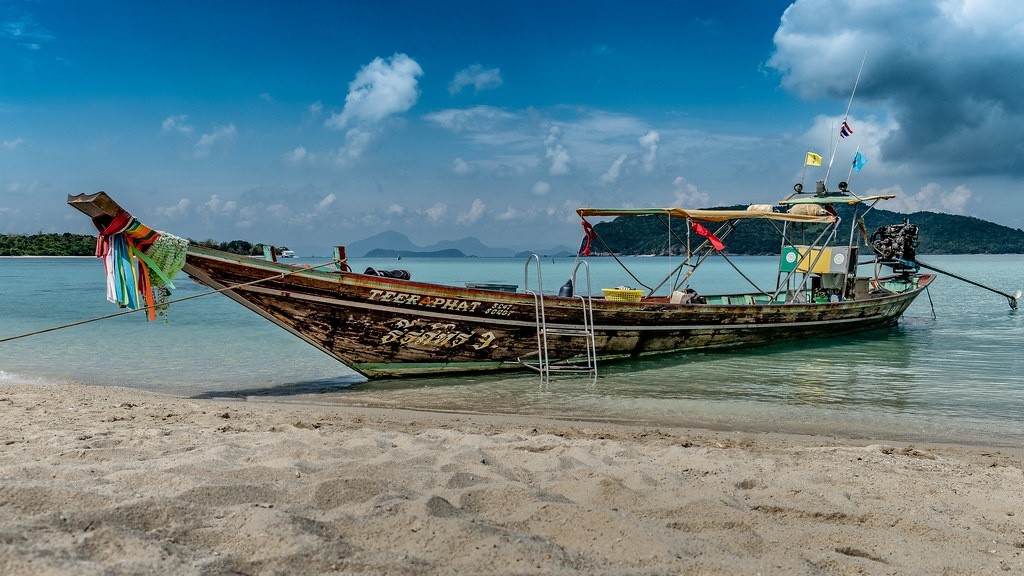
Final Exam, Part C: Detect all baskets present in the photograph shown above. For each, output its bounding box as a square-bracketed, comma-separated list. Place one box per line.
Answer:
[601, 288, 644, 302]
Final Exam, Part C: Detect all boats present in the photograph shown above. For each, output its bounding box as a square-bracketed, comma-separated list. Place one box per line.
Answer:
[281, 251, 295, 258]
[67, 181, 938, 381]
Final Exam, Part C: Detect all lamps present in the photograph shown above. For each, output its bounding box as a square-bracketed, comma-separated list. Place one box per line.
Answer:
[838, 182, 848, 192]
[794, 184, 802, 194]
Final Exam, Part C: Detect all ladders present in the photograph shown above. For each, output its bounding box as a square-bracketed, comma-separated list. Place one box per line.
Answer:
[517, 254, 598, 377]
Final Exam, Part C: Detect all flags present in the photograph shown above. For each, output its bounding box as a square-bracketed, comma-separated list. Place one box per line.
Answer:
[852, 151, 867, 172]
[841, 121, 854, 138]
[806, 152, 822, 166]
